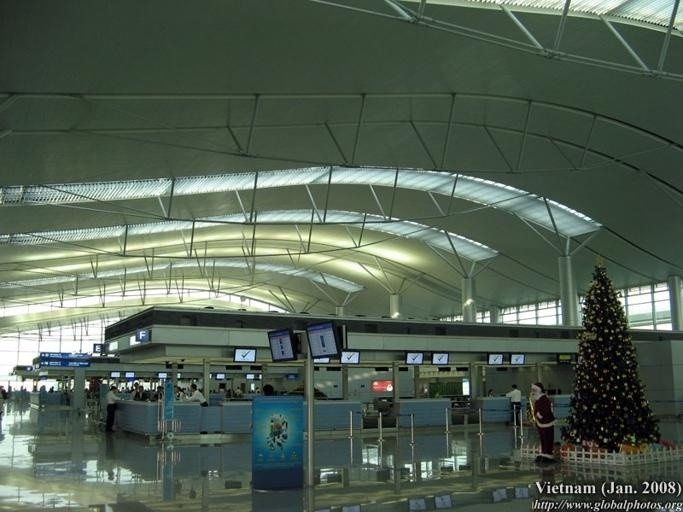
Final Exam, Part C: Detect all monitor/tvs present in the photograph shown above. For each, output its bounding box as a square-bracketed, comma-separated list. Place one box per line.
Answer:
[267, 327, 298, 363]
[340, 351, 361, 364]
[488, 353, 504, 367]
[431, 353, 450, 365]
[342, 504, 361, 512]
[510, 354, 525, 365]
[433, 495, 454, 510]
[216, 373, 226, 380]
[406, 352, 423, 365]
[407, 497, 427, 511]
[125, 372, 136, 377]
[514, 485, 529, 499]
[314, 357, 331, 365]
[109, 372, 121, 378]
[305, 319, 343, 359]
[232, 347, 257, 363]
[158, 373, 167, 380]
[246, 373, 255, 379]
[176, 372, 182, 379]
[491, 488, 508, 501]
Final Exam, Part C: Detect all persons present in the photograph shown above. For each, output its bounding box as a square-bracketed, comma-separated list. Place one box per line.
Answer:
[504, 383, 522, 429]
[528, 383, 557, 464]
[104, 386, 121, 433]
[121, 382, 275, 437]
[0, 382, 101, 416]
[486, 388, 497, 398]
